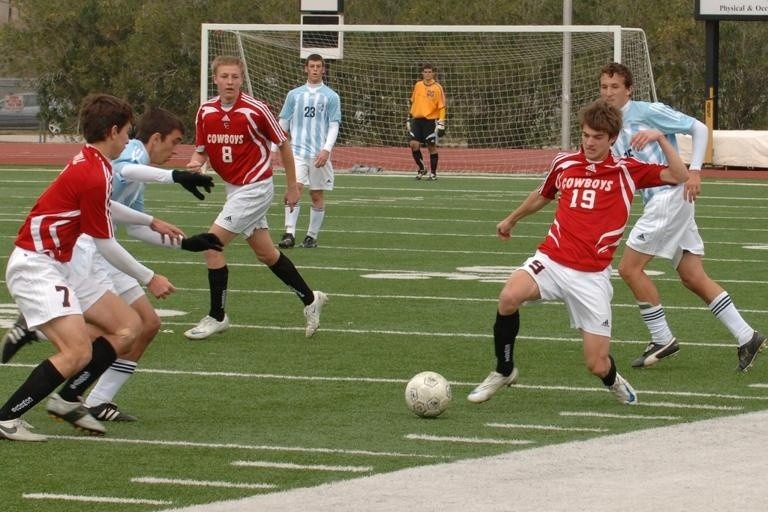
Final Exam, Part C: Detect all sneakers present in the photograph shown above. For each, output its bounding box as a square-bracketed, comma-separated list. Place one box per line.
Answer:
[735, 329, 767, 375]
[467, 367, 517, 404]
[632, 335, 681, 367]
[300, 236, 317, 247]
[608, 372, 637, 406]
[430, 173, 437, 181]
[416, 168, 427, 181]
[279, 233, 295, 249]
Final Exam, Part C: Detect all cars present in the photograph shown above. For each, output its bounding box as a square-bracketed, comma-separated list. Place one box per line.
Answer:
[1, 92, 75, 134]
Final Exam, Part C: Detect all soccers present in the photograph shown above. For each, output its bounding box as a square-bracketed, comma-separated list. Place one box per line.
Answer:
[405, 371, 450, 416]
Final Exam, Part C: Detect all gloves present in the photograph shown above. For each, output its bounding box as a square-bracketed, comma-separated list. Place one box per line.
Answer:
[173, 169, 215, 200]
[436, 121, 445, 137]
[180, 232, 225, 254]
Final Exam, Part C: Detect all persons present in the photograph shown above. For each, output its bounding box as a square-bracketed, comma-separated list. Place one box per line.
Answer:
[185, 55, 328, 339]
[0, 89, 184, 440]
[464, 97, 690, 403]
[600, 61, 768, 375]
[405, 65, 447, 180]
[0, 105, 223, 358]
[271, 54, 341, 248]
